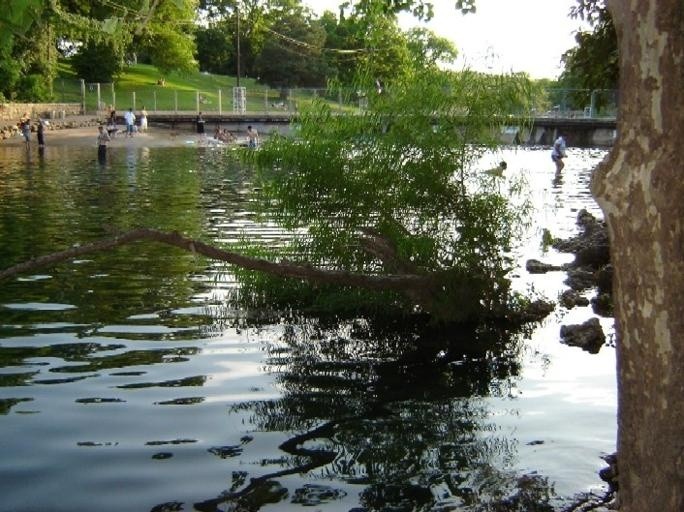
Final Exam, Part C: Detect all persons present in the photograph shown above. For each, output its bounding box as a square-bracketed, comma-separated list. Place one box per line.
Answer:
[23, 119, 31, 152]
[214, 125, 237, 143]
[484, 160, 507, 177]
[245, 126, 258, 151]
[97, 125, 109, 166]
[550, 131, 568, 186]
[123, 107, 135, 137]
[269, 101, 287, 111]
[140, 105, 147, 137]
[195, 112, 204, 134]
[107, 105, 117, 130]
[37, 120, 43, 149]
